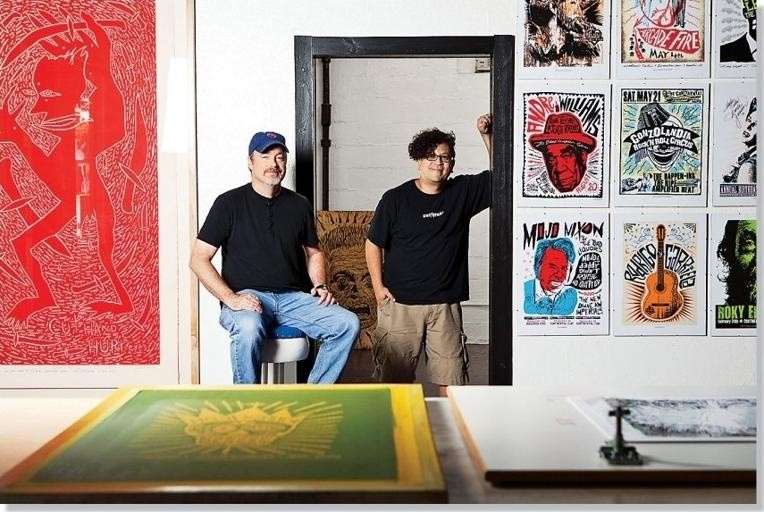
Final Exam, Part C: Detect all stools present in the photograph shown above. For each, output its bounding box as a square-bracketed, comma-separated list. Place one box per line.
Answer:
[261, 321, 310, 384]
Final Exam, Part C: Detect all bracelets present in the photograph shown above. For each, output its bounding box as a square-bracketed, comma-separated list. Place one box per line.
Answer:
[315, 284, 329, 289]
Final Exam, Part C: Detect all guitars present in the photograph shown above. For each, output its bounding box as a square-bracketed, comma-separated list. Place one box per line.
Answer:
[640, 224, 684, 322]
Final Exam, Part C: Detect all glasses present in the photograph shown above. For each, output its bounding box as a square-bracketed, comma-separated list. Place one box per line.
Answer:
[426, 153, 454, 162]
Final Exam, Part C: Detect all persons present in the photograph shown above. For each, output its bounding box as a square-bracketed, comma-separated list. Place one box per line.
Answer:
[320, 222, 378, 349]
[524, 237, 577, 315]
[717, 220, 756, 318]
[720, 0, 756, 63]
[722, 99, 757, 184]
[364, 113, 491, 397]
[531, 113, 596, 192]
[189, 131, 360, 384]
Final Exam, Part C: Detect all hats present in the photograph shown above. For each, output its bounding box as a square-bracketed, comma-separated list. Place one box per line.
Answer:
[249, 131, 289, 155]
[640, 103, 670, 127]
[528, 113, 597, 152]
